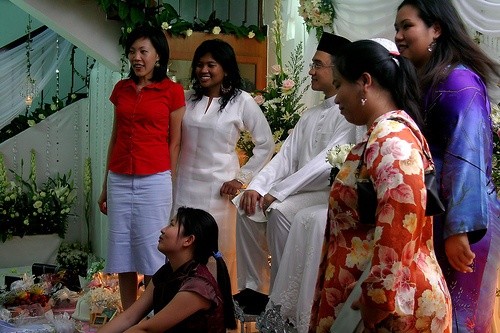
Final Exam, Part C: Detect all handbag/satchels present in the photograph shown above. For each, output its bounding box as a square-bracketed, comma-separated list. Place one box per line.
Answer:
[356, 168, 446, 229]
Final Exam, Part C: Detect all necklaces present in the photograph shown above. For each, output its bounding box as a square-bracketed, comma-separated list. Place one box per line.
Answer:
[139, 88, 141, 91]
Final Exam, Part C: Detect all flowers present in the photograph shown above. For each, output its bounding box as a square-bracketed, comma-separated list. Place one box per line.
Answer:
[238, 0, 312, 161]
[0, 148, 78, 243]
[325, 143, 356, 186]
[97, 0, 265, 43]
[27, 94, 77, 127]
[489, 102, 500, 192]
[298, 0, 337, 43]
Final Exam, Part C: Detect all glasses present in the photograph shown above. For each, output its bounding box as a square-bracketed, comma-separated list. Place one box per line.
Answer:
[308, 63, 336, 70]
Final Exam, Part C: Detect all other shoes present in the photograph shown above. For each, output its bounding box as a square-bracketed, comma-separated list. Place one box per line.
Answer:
[233, 284, 269, 307]
[242, 296, 270, 315]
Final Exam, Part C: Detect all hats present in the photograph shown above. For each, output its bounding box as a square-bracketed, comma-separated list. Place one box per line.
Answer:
[317, 31, 353, 56]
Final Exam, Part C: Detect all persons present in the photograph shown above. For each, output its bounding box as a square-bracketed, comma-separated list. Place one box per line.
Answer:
[304, 40, 453, 333]
[167, 39, 275, 299]
[231, 33, 367, 333]
[98, 26, 186, 312]
[99, 206, 237, 333]
[393, 0, 500, 333]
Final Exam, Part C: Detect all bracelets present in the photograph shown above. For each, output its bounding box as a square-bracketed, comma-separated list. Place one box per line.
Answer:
[233, 178, 243, 185]
